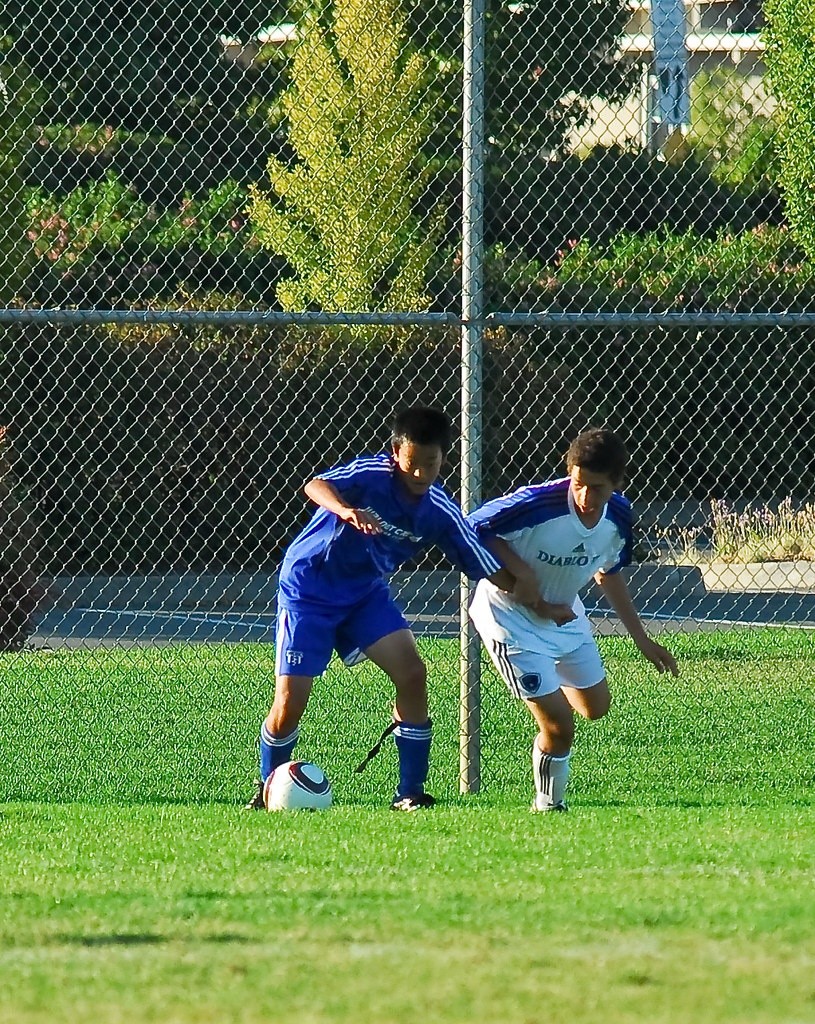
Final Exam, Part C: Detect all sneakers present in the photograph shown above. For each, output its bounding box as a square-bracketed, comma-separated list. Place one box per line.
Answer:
[245, 782, 265, 810]
[389, 794, 433, 812]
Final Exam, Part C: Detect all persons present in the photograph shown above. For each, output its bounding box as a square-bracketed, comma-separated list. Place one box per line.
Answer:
[470, 429, 679, 812]
[246, 407, 578, 809]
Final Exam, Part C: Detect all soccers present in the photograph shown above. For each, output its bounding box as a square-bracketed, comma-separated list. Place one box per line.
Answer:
[262, 760, 333, 817]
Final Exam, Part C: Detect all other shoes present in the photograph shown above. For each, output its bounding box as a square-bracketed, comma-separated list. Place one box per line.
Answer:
[528, 801, 568, 814]
